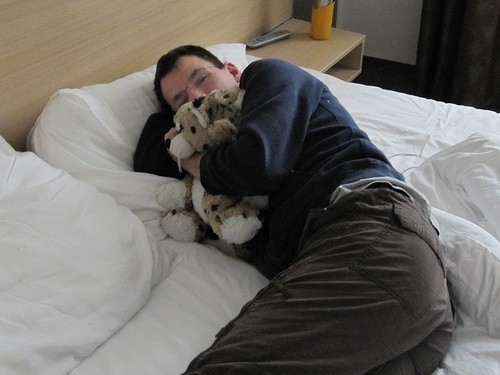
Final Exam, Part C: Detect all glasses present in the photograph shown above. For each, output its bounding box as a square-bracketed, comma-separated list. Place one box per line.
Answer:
[173, 64, 224, 111]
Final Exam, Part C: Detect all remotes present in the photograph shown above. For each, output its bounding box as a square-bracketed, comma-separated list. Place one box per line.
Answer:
[245, 30, 290, 48]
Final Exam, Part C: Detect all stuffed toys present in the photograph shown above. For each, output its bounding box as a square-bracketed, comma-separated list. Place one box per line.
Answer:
[162, 88, 269, 245]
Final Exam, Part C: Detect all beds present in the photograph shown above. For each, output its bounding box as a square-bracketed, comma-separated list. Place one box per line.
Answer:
[0, 1, 500, 375]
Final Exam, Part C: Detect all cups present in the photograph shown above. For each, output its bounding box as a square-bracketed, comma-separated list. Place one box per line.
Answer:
[310, 0, 335, 40]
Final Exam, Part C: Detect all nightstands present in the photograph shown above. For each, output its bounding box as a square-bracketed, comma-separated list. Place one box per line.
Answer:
[248, 17, 366, 81]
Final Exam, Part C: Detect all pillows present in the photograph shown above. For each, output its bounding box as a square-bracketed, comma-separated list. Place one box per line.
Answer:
[0, 134, 185, 375]
[26, 42, 248, 241]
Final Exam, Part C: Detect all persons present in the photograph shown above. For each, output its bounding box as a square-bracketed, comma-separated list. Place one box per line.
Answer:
[132, 45, 456, 375]
